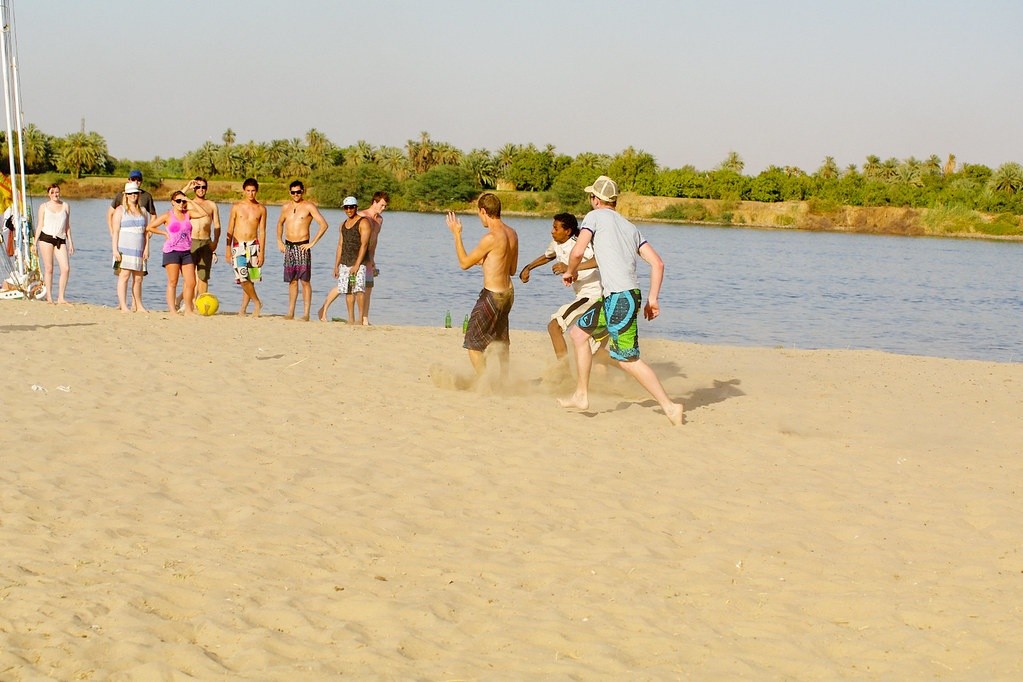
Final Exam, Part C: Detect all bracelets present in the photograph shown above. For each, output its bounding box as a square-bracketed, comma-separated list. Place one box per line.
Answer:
[32, 244, 37, 247]
[213, 253, 216, 255]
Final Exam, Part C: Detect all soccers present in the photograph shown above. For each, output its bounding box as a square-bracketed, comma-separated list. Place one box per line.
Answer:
[196, 293, 219, 316]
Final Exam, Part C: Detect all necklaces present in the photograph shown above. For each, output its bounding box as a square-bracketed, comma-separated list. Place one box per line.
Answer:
[291, 200, 304, 213]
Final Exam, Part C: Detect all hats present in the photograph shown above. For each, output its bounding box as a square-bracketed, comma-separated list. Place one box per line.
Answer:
[130, 170, 143, 178]
[341, 196, 358, 207]
[124, 182, 142, 194]
[5, 214, 14, 231]
[5, 270, 26, 286]
[584, 176, 618, 202]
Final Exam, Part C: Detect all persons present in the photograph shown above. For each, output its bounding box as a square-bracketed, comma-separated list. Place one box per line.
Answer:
[107, 170, 157, 312]
[2, 189, 43, 283]
[332, 196, 371, 326]
[226, 177, 267, 318]
[30, 184, 74, 306]
[317, 191, 390, 326]
[446, 194, 518, 396]
[146, 191, 209, 316]
[519, 212, 627, 394]
[174, 251, 218, 311]
[112, 182, 149, 312]
[277, 179, 329, 322]
[557, 176, 683, 426]
[181, 176, 221, 314]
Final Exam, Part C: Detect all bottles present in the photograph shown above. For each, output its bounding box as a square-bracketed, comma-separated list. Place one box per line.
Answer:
[348, 274, 355, 293]
[463, 314, 469, 334]
[445, 310, 452, 328]
[113, 252, 123, 270]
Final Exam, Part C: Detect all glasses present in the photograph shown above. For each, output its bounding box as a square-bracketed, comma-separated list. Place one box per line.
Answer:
[172, 199, 187, 203]
[344, 205, 357, 209]
[194, 185, 207, 189]
[589, 194, 601, 201]
[126, 192, 139, 195]
[129, 177, 142, 182]
[290, 189, 304, 194]
